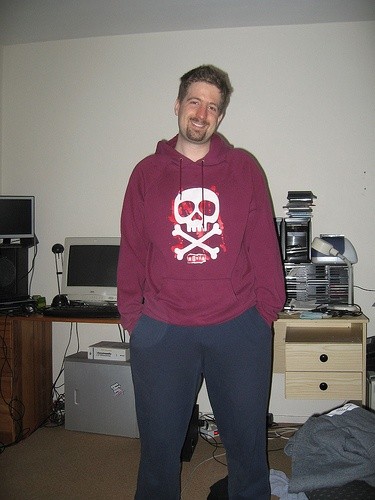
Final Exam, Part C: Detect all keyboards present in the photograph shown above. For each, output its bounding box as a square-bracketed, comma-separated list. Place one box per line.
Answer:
[43, 305, 121, 319]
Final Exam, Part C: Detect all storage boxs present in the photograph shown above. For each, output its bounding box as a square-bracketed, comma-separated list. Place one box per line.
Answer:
[88, 341, 130, 361]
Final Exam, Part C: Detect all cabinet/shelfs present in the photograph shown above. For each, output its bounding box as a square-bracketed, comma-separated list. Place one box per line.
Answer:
[0, 319, 52, 443]
[284, 326, 363, 401]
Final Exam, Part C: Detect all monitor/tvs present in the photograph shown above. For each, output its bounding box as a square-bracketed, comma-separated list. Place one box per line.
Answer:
[0, 196, 35, 239]
[60, 237, 121, 307]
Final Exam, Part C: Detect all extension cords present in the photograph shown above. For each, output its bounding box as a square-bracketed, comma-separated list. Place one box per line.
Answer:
[200, 424, 219, 439]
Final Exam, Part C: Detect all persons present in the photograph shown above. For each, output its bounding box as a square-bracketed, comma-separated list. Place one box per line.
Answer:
[116, 67, 286, 500]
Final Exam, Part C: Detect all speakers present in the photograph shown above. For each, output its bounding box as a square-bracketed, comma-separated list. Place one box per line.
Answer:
[180, 405, 200, 463]
[0, 244, 29, 304]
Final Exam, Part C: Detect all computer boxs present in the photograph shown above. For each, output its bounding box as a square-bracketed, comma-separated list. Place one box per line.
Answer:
[63, 351, 142, 438]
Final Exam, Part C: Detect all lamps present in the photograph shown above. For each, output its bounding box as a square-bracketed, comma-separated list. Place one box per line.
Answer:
[310, 238, 360, 312]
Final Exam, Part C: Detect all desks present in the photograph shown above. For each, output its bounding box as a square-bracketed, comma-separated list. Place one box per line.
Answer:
[0, 304, 369, 442]
